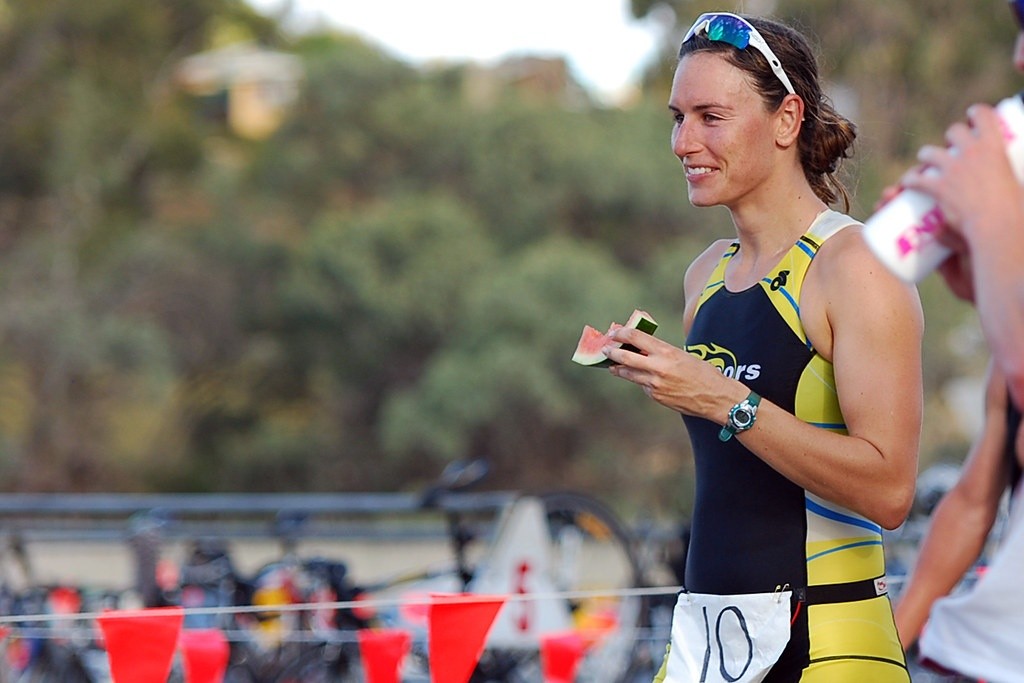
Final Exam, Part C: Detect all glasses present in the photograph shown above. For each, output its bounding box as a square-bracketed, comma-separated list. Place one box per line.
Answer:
[679, 11, 806, 121]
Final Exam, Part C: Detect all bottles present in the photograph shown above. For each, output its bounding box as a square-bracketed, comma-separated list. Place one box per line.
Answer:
[859, 96, 1024, 283]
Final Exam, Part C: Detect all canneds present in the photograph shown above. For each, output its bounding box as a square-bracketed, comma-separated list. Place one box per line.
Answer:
[859, 93, 1024, 282]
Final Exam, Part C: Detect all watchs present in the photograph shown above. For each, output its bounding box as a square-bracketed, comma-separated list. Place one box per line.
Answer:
[720, 391, 761, 441]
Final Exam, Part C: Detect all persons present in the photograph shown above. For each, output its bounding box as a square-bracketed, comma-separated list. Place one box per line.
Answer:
[894, 0, 1024, 683]
[602, 16, 925, 683]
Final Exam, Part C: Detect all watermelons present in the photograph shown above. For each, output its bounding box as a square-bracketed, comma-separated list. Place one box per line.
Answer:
[572, 309, 658, 368]
